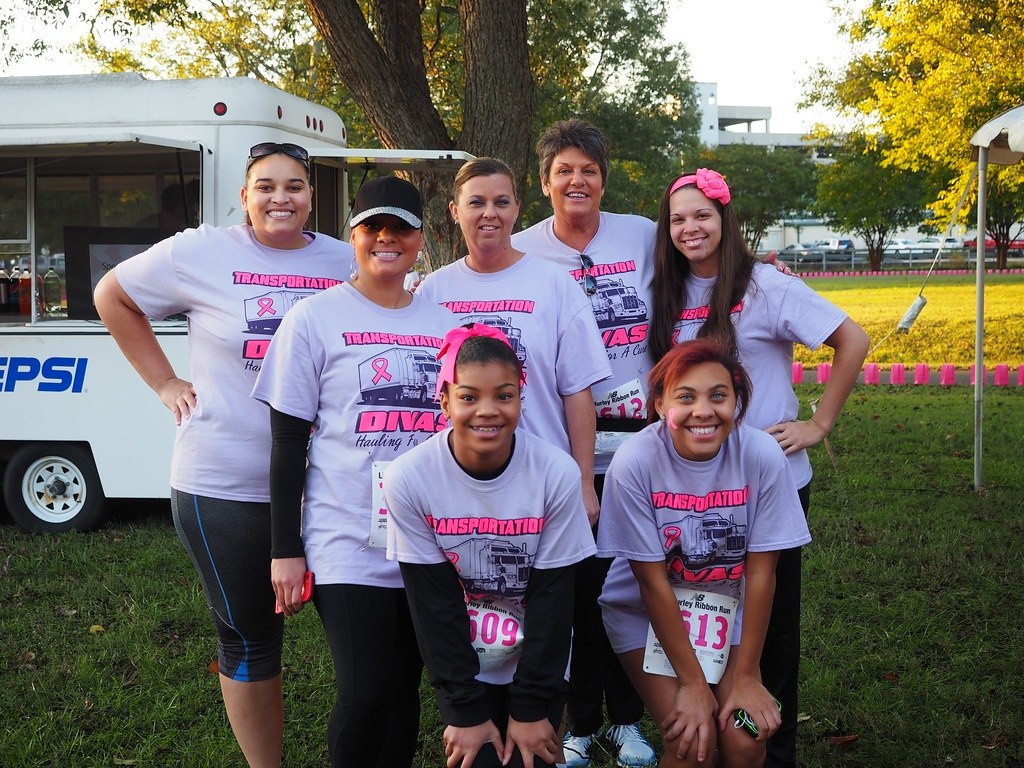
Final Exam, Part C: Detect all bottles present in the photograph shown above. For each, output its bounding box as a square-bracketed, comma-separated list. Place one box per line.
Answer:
[0, 267, 44, 316]
[43, 267, 61, 313]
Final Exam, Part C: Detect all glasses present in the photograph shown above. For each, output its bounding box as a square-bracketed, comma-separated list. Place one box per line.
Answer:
[250, 142, 309, 167]
[579, 253, 598, 296]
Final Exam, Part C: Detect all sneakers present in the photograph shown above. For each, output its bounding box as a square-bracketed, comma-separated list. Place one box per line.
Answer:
[555, 730, 596, 768]
[605, 720, 657, 768]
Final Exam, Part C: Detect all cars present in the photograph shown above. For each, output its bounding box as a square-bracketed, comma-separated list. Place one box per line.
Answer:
[878, 238, 924, 260]
[913, 236, 964, 259]
[778, 238, 855, 262]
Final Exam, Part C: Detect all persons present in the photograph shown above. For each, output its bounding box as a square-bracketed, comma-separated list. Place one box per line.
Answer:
[137, 179, 199, 243]
[597, 336, 814, 768]
[647, 169, 871, 768]
[383, 322, 599, 768]
[250, 175, 460, 768]
[93, 143, 353, 768]
[414, 158, 610, 759]
[410, 116, 794, 768]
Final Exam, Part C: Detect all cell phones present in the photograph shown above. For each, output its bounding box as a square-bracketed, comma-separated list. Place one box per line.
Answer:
[733, 697, 782, 739]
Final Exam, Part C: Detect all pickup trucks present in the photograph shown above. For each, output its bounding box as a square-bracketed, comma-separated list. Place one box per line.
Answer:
[963, 230, 1024, 258]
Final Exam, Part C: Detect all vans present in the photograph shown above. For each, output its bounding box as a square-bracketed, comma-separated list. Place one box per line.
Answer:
[0, 70, 479, 536]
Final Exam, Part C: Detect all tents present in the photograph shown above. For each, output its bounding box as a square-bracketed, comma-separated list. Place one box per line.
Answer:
[897, 107, 1022, 488]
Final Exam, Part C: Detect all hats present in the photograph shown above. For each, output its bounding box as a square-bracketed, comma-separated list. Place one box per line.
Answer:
[349, 175, 424, 229]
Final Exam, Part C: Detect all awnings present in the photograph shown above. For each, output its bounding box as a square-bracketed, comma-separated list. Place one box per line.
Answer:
[0, 132, 200, 232]
[298, 146, 477, 238]
